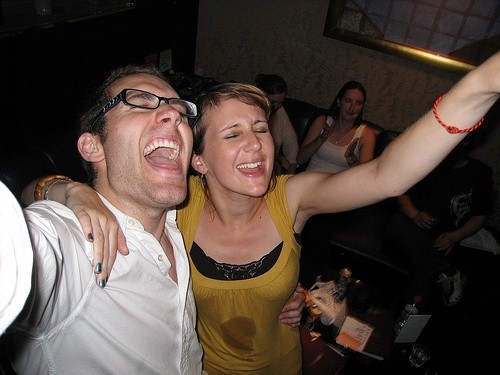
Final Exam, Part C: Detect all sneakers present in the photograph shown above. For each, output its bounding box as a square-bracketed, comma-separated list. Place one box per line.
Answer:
[393, 302, 419, 336]
[435, 265, 462, 307]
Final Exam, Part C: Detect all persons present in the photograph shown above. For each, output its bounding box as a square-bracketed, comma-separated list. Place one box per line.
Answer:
[297, 81, 378, 283]
[256, 74, 298, 175]
[366, 133, 492, 318]
[20, 52, 500, 375]
[0, 64, 307, 375]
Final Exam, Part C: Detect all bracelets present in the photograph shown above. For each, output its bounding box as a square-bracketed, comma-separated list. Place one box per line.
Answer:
[34, 175, 71, 201]
[44, 179, 68, 200]
[433, 96, 483, 133]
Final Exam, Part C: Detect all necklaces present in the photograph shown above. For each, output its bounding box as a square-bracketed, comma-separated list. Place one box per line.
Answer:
[335, 130, 351, 144]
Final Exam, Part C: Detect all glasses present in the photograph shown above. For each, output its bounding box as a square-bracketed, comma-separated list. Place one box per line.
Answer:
[89, 88, 201, 130]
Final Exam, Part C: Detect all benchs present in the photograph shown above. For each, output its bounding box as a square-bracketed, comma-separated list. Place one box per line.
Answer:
[280, 97, 493, 300]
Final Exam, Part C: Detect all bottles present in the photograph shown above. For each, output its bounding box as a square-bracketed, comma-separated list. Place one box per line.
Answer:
[393, 295, 421, 338]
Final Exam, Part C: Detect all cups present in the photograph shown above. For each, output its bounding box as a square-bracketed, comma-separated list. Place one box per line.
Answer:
[409, 344, 431, 368]
